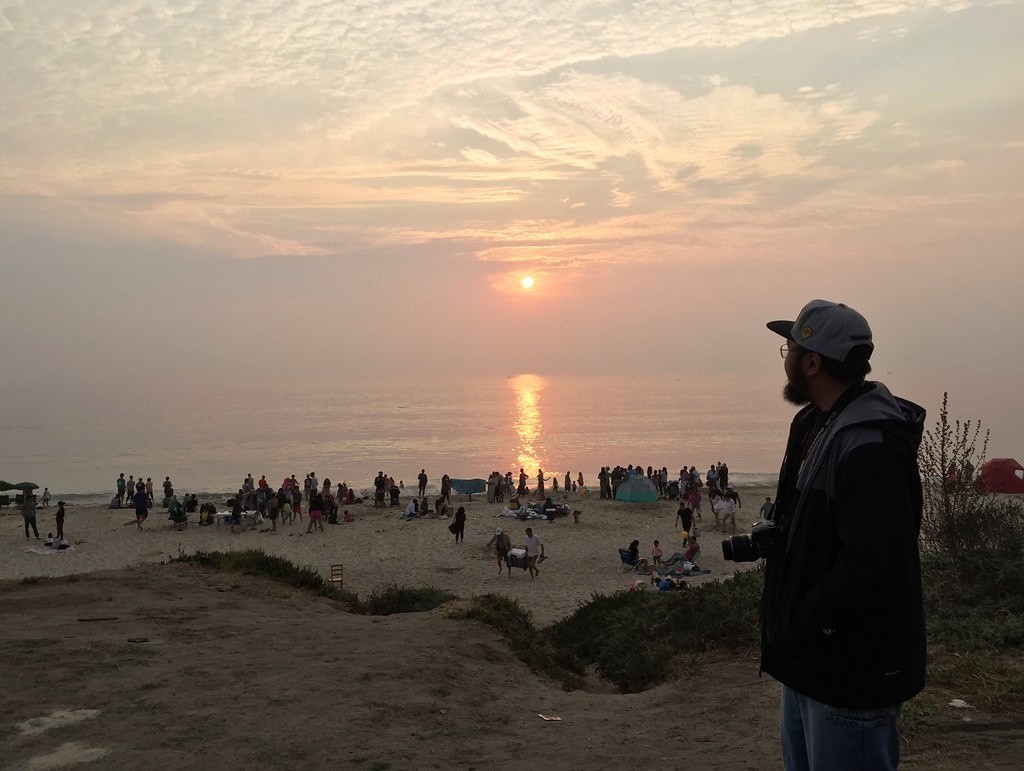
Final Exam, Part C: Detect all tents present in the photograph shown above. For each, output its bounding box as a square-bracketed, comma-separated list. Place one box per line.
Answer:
[975, 457, 1024, 492]
[615, 473, 659, 503]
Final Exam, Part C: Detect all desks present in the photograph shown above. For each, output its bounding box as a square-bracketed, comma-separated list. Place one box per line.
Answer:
[217, 511, 257, 530]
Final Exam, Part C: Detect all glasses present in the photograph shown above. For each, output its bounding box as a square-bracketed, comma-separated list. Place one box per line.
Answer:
[780, 344, 808, 358]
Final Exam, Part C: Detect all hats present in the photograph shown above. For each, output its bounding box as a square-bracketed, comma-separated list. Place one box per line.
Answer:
[496, 527, 503, 535]
[766, 300, 874, 363]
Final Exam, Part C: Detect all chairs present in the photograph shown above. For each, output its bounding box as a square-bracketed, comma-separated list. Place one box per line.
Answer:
[617, 548, 647, 572]
[0, 495, 37, 510]
[668, 546, 702, 574]
[327, 563, 343, 591]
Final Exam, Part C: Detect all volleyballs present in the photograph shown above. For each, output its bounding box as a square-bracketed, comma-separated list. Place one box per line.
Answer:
[681, 530, 688, 538]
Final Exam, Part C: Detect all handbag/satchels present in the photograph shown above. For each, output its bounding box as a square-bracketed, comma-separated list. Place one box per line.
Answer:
[690, 527, 700, 536]
[714, 496, 725, 511]
[449, 523, 457, 535]
[258, 514, 264, 522]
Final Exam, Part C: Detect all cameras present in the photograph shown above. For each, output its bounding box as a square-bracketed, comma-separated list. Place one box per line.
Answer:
[721, 523, 775, 563]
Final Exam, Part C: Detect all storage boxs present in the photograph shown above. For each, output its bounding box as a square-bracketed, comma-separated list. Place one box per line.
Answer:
[507, 548, 527, 568]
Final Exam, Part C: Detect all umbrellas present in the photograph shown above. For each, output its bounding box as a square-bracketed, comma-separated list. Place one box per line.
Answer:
[14, 482, 39, 494]
[0, 481, 16, 491]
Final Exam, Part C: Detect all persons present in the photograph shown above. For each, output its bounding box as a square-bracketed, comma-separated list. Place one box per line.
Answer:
[53, 501, 66, 541]
[21, 495, 42, 540]
[763, 297, 926, 771]
[948, 460, 974, 493]
[42, 488, 52, 507]
[108, 460, 776, 592]
